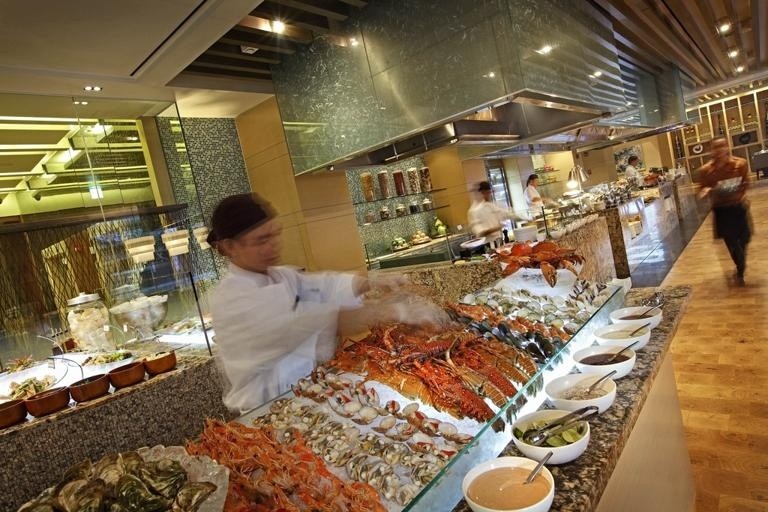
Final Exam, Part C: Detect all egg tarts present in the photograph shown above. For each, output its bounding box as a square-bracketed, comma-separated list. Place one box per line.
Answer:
[488, 239, 587, 288]
[182, 415, 386, 511]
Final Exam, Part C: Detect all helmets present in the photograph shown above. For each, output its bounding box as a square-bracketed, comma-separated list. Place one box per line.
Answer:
[513, 225, 539, 245]
[628, 221, 642, 239]
[460, 237, 486, 248]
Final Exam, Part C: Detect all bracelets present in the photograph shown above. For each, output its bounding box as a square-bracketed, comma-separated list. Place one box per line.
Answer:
[716, 176, 743, 190]
[142, 348, 178, 377]
[536, 232, 547, 241]
[571, 344, 637, 381]
[0, 399, 28, 430]
[108, 361, 146, 390]
[549, 213, 599, 240]
[68, 373, 110, 403]
[594, 201, 606, 212]
[609, 306, 663, 331]
[510, 409, 591, 465]
[462, 455, 556, 512]
[24, 385, 70, 418]
[591, 323, 651, 352]
[543, 372, 617, 415]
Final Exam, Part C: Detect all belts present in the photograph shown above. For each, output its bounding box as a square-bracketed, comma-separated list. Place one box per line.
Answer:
[112, 300, 169, 344]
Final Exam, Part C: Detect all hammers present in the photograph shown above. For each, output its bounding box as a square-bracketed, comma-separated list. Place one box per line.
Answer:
[325, 318, 544, 433]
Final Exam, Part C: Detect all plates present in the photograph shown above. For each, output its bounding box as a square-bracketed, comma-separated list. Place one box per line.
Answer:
[535, 169, 562, 186]
[352, 187, 450, 227]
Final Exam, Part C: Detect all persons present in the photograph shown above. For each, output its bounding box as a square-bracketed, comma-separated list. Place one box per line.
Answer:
[466, 183, 530, 249]
[206, 192, 448, 413]
[625, 156, 645, 188]
[522, 175, 544, 220]
[696, 136, 758, 287]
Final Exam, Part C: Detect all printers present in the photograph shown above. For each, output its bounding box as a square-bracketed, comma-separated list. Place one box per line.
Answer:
[521, 405, 599, 447]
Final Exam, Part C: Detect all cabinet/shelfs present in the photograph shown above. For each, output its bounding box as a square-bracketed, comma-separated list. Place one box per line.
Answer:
[419, 165, 434, 193]
[359, 172, 375, 202]
[376, 170, 393, 201]
[395, 202, 409, 217]
[406, 166, 422, 195]
[379, 205, 392, 221]
[65, 290, 111, 354]
[364, 208, 377, 224]
[408, 200, 421, 214]
[422, 197, 433, 211]
[392, 170, 409, 198]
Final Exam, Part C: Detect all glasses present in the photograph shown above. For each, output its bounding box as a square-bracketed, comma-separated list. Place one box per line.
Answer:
[566, 164, 589, 192]
[125, 210, 211, 264]
